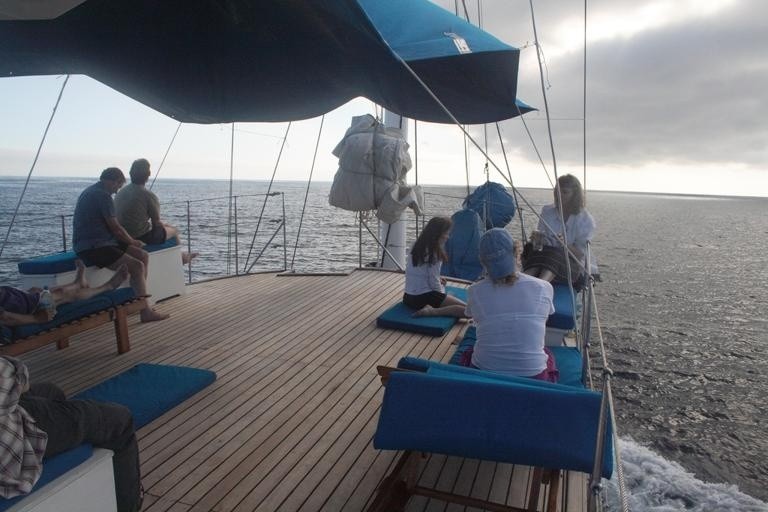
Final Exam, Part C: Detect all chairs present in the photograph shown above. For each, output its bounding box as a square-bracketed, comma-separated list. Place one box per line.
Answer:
[0, 283, 146, 357]
[361, 327, 616, 510]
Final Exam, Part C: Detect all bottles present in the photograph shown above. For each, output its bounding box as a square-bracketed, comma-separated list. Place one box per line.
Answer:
[39, 286, 54, 310]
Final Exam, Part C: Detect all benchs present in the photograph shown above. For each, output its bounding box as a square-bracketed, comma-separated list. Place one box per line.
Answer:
[18, 236, 185, 318]
[544, 283, 581, 347]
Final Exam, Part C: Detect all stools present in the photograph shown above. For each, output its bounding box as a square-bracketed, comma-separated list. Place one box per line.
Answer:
[0, 443, 117, 511]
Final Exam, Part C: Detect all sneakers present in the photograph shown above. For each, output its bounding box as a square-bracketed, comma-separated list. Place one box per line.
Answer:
[140, 307, 170, 323]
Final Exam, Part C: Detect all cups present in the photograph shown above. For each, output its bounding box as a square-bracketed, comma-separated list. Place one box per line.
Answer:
[531, 230, 545, 252]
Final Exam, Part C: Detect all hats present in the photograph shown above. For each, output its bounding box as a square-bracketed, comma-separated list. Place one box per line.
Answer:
[478, 227, 517, 281]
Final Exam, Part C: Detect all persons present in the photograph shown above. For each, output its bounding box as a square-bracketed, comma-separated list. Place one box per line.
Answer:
[70, 165, 170, 323]
[0, 355, 143, 512]
[113, 158, 198, 265]
[462, 227, 559, 384]
[520, 174, 595, 282]
[402, 214, 468, 318]
[0, 258, 127, 330]
